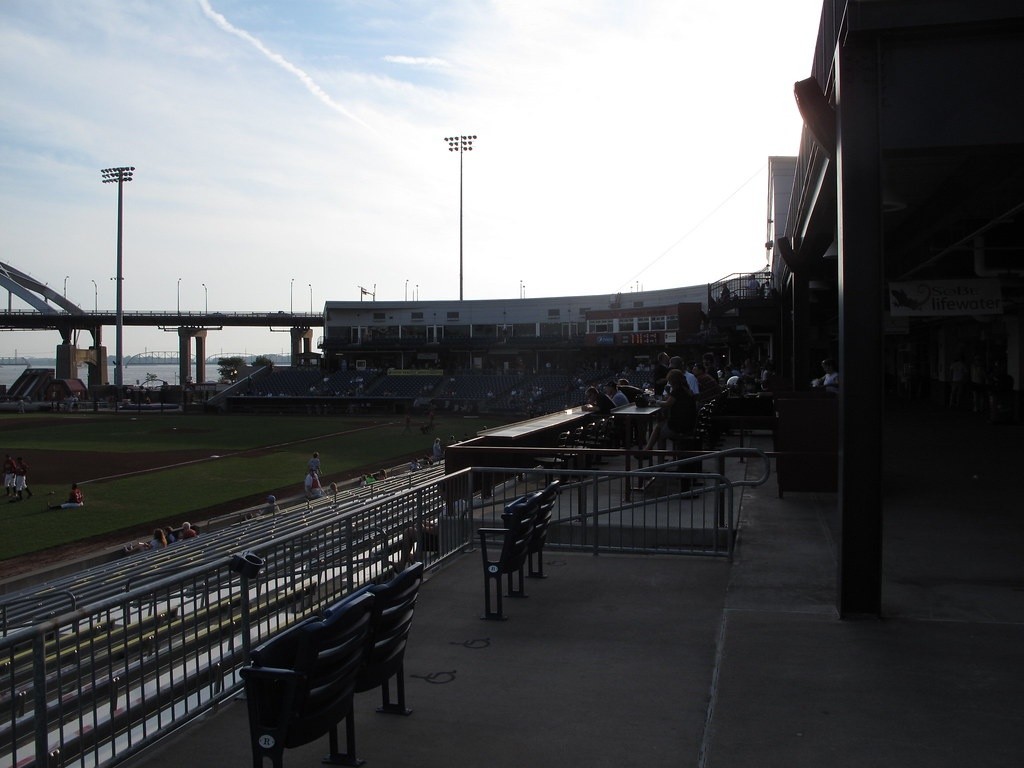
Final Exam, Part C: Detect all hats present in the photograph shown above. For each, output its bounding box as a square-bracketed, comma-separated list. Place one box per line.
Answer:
[266, 495, 275, 501]
[309, 468, 316, 471]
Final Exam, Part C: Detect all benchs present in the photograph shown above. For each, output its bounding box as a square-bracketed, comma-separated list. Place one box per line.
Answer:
[0, 459, 445, 768]
[668, 388, 730, 468]
[478, 415, 615, 621]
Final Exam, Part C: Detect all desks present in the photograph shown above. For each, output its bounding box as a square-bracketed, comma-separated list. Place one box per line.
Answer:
[610, 402, 662, 502]
[476, 403, 596, 484]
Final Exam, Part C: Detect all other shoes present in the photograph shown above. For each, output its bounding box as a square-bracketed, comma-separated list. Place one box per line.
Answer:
[634, 449, 653, 460]
[123, 545, 128, 555]
[48, 503, 51, 511]
[4, 493, 34, 500]
[129, 543, 134, 551]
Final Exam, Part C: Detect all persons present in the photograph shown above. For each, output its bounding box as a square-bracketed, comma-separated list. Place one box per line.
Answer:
[937, 360, 1015, 423]
[124, 521, 200, 556]
[411, 456, 433, 472]
[256, 495, 279, 517]
[56, 401, 62, 414]
[235, 369, 494, 400]
[2, 454, 34, 501]
[509, 386, 550, 411]
[391, 496, 468, 573]
[47, 483, 84, 510]
[360, 469, 387, 487]
[810, 358, 839, 388]
[433, 438, 443, 457]
[463, 433, 471, 441]
[17, 398, 25, 414]
[584, 352, 783, 460]
[447, 435, 457, 446]
[399, 411, 435, 436]
[305, 453, 339, 499]
